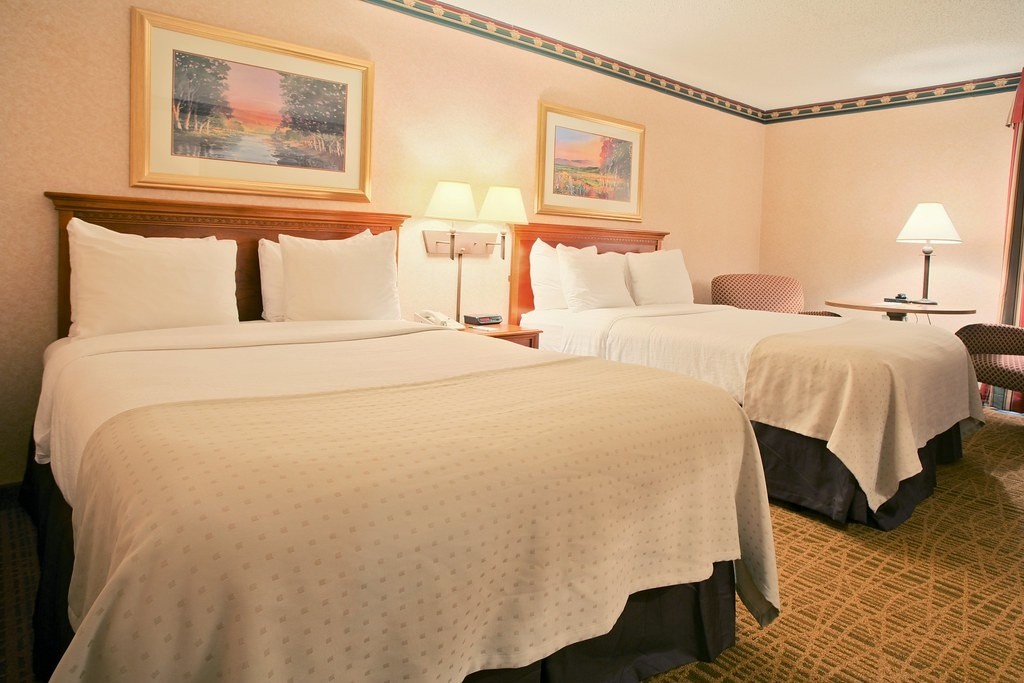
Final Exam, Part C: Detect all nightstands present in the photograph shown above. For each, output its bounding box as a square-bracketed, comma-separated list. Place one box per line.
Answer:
[460, 320, 543, 351]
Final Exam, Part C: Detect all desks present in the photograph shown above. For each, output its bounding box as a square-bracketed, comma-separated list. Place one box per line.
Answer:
[824, 299, 976, 320]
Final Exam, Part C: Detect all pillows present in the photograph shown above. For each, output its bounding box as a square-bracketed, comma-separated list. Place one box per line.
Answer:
[65, 217, 400, 337]
[528, 237, 696, 311]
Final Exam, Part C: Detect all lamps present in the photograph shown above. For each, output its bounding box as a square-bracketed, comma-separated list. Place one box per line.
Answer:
[424, 182, 529, 322]
[895, 201, 962, 305]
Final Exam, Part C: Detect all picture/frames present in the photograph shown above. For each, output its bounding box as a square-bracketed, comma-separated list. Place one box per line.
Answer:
[128, 5, 376, 206]
[536, 99, 646, 222]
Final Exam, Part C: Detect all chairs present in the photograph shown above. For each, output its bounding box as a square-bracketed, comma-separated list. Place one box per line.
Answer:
[952, 321, 1024, 393]
[711, 274, 840, 318]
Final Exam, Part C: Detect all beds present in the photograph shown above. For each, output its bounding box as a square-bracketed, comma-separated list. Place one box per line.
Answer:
[16, 190, 780, 682]
[509, 221, 986, 534]
